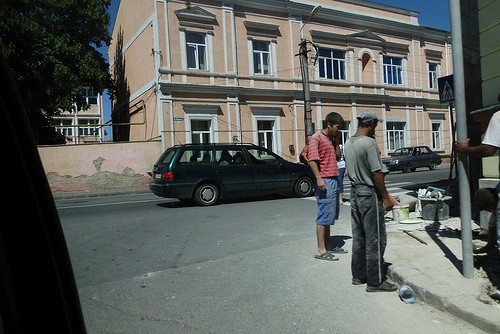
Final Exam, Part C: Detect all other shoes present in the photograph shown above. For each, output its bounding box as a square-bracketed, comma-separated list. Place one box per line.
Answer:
[365, 282, 398, 292]
[352, 276, 368, 285]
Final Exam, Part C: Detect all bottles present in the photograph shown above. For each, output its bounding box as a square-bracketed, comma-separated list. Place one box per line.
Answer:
[398, 284, 417, 303]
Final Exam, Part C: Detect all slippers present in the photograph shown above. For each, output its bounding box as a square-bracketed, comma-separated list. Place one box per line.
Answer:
[314, 251, 339, 261]
[325, 244, 347, 254]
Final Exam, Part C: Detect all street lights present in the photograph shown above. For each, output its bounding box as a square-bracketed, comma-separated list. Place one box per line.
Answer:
[299, 4, 323, 136]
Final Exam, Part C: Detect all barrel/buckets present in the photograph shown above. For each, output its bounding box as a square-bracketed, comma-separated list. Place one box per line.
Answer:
[392, 204, 410, 221]
[420, 188, 452, 220]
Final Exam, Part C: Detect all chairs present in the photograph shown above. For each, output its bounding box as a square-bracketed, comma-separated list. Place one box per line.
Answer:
[233, 152, 245, 163]
[190, 155, 197, 162]
[218, 151, 232, 163]
[202, 154, 210, 162]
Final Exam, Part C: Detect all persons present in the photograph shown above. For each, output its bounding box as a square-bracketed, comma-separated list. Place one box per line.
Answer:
[336, 144, 346, 204]
[344, 112, 399, 293]
[308, 112, 348, 262]
[300, 136, 311, 165]
[457, 111, 500, 157]
[471, 188, 500, 253]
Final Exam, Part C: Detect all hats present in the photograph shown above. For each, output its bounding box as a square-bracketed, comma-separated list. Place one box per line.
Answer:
[356, 110, 383, 123]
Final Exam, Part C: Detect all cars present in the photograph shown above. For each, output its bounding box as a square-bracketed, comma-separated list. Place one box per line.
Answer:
[149, 142, 316, 207]
[382, 145, 443, 173]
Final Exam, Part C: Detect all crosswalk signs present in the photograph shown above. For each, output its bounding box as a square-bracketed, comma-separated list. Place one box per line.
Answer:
[438, 73, 455, 104]
